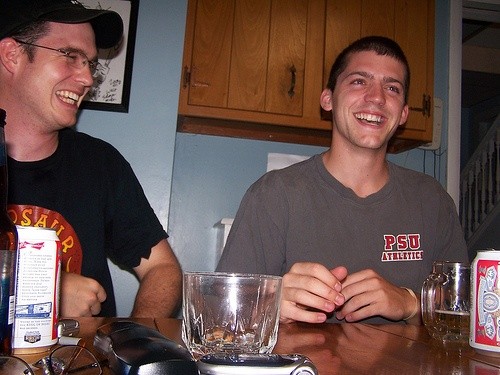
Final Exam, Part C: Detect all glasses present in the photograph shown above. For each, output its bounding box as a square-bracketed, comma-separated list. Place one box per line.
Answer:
[12, 36, 103, 79]
[1, 339, 110, 375]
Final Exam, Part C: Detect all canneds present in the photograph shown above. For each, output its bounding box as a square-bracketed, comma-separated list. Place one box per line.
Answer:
[8, 224, 61, 355]
[468, 249, 500, 359]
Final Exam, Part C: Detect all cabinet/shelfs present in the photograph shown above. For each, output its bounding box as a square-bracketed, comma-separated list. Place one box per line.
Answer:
[176, 0, 437, 154]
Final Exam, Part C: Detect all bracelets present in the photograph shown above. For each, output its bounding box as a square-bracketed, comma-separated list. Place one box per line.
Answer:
[399, 286, 418, 321]
[406, 325, 417, 348]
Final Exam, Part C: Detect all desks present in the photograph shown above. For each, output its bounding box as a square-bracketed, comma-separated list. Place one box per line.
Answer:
[0, 315, 500, 375]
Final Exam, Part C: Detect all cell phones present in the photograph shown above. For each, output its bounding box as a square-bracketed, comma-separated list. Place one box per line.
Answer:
[56, 318, 80, 337]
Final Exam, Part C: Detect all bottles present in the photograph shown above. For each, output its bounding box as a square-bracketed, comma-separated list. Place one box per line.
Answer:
[0, 109, 21, 367]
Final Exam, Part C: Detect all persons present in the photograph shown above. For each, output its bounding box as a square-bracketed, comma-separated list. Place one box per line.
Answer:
[270, 324, 420, 375]
[206, 36, 471, 324]
[0, 0, 186, 320]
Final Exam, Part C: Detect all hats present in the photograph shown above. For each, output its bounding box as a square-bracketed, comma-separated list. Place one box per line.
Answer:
[0, 1, 124, 48]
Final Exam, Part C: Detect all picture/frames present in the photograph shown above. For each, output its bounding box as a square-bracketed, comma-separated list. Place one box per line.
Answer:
[76, 0, 140, 113]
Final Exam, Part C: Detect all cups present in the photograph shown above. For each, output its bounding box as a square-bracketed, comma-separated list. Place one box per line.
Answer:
[181, 271, 283, 360]
[420, 259, 471, 345]
